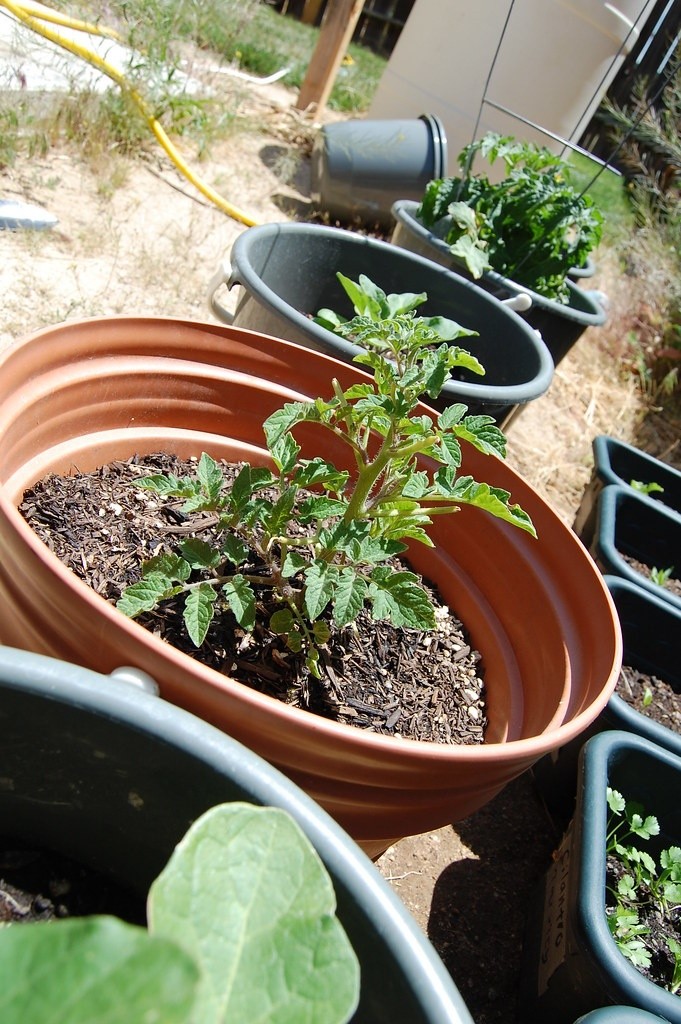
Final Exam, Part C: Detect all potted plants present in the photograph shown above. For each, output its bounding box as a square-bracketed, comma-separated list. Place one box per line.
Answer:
[0, 131, 681, 1024]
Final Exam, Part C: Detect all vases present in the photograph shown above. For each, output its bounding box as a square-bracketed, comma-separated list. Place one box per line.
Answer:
[310, 113, 447, 223]
